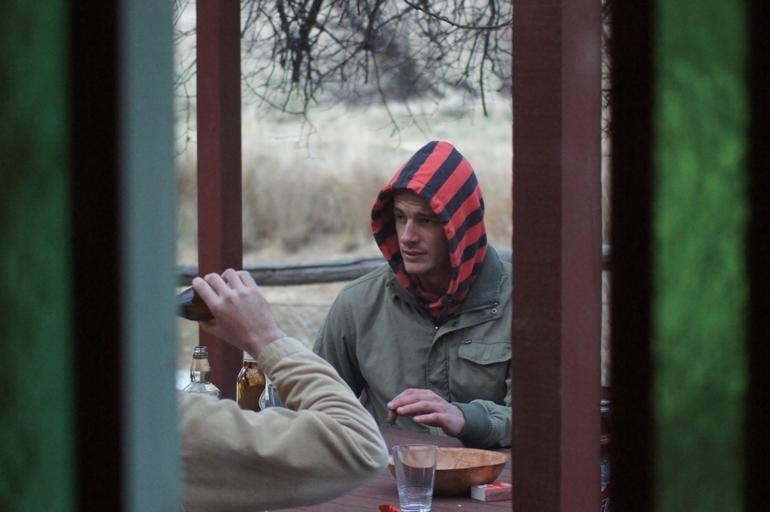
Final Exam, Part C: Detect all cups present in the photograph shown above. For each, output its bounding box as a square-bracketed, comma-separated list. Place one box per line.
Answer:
[393, 446, 436, 511]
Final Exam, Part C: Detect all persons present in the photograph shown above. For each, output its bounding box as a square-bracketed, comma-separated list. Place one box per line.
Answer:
[172, 265, 388, 512]
[311, 140, 512, 447]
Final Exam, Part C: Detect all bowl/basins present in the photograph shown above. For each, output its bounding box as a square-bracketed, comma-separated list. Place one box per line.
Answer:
[388, 448, 510, 495]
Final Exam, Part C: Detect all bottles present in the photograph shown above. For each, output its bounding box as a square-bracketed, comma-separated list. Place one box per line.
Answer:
[174, 287, 214, 322]
[182, 345, 284, 412]
